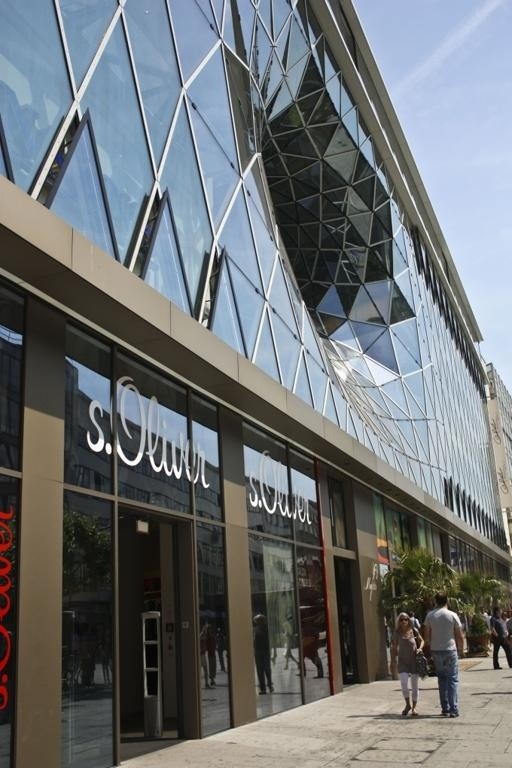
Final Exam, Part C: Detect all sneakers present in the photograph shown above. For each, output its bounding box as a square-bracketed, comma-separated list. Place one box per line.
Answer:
[442, 711, 459, 718]
[259, 686, 275, 695]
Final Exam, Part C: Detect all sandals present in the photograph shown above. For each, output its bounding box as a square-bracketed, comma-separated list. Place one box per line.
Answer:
[402, 704, 419, 715]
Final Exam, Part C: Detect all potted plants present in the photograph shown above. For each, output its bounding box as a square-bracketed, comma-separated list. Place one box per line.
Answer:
[455, 573, 508, 653]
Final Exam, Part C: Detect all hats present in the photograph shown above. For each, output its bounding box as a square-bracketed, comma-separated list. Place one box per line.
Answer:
[253, 614, 267, 625]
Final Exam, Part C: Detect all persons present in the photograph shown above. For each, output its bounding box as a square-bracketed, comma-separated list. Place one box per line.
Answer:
[199, 614, 324, 696]
[409, 611, 421, 631]
[483, 606, 512, 648]
[98, 626, 111, 687]
[489, 606, 511, 670]
[422, 592, 465, 719]
[391, 612, 424, 717]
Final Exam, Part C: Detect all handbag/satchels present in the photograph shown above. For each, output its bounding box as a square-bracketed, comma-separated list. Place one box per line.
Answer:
[415, 652, 429, 677]
